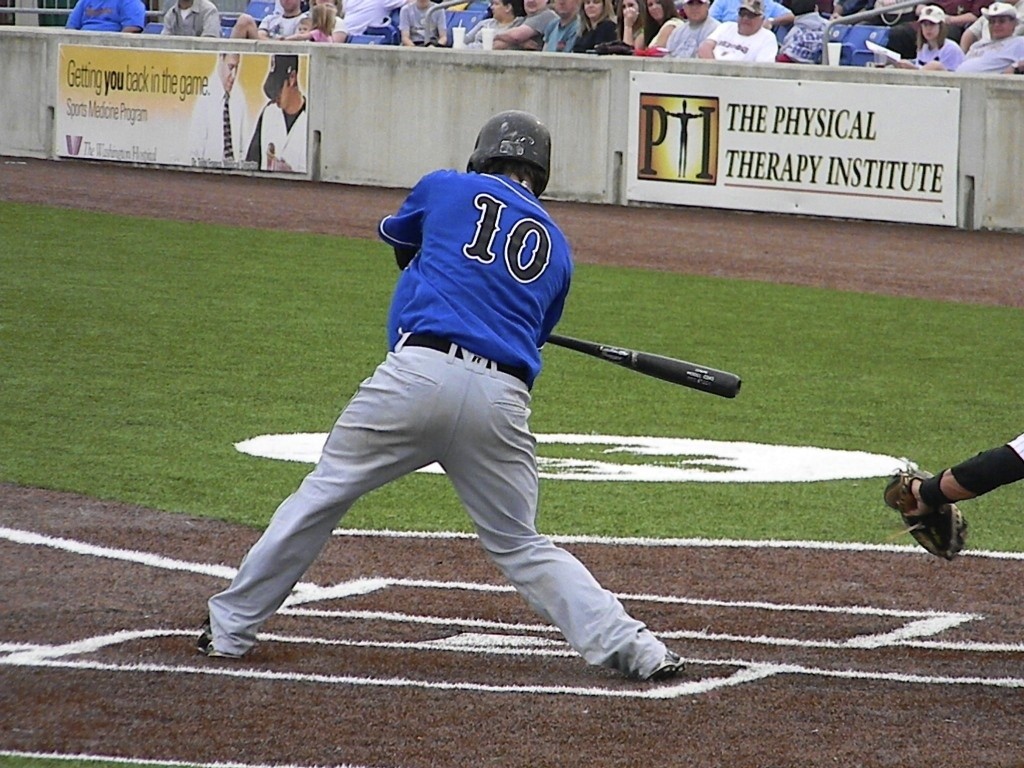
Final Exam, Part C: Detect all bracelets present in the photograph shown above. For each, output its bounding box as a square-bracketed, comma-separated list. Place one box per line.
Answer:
[769, 18, 775, 24]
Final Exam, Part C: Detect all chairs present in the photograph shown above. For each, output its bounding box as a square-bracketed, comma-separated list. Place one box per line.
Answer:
[139, 0, 893, 67]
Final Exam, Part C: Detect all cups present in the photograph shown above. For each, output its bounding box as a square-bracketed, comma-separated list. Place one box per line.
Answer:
[481, 28, 494, 51]
[451, 27, 466, 48]
[826, 43, 842, 66]
[66, 135, 82, 155]
[873, 50, 886, 67]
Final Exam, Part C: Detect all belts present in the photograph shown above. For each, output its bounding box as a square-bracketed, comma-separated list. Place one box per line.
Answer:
[402, 332, 529, 384]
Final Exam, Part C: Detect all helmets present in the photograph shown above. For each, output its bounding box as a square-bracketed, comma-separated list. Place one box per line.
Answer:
[467, 110, 551, 198]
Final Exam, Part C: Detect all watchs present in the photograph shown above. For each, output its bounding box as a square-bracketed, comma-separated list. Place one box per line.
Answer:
[1012, 63, 1020, 73]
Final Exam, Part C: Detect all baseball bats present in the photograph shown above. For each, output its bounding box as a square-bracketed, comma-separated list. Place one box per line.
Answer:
[546, 332, 742, 400]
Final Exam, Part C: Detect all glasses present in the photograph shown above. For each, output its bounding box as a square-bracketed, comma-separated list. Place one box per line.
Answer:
[622, 3, 634, 8]
[920, 23, 936, 28]
[686, 1, 703, 6]
[739, 10, 757, 18]
[989, 18, 1007, 24]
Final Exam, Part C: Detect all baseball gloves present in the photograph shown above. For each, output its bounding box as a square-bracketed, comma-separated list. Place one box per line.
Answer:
[883, 465, 971, 564]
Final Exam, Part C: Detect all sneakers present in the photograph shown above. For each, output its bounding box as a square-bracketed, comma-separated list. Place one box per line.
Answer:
[197, 616, 244, 659]
[645, 645, 686, 680]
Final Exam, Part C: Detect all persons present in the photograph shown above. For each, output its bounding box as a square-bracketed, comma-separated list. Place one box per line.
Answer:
[161, 0, 222, 38]
[541, 0, 584, 52]
[697, 0, 778, 62]
[464, 0, 560, 50]
[709, 0, 794, 30]
[889, 0, 1024, 59]
[667, 0, 722, 58]
[65, 0, 145, 33]
[243, 55, 306, 162]
[922, 3, 1024, 73]
[229, 0, 408, 44]
[196, 108, 686, 677]
[571, 0, 687, 54]
[783, 0, 932, 25]
[399, 0, 448, 47]
[884, 433, 1024, 563]
[893, 6, 964, 71]
[190, 54, 246, 160]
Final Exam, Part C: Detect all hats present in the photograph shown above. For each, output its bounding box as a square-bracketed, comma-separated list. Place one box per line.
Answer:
[981, 2, 1015, 18]
[918, 5, 945, 23]
[264, 55, 298, 98]
[740, 0, 764, 15]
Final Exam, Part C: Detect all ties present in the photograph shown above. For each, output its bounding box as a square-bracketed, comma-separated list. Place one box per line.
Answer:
[223, 91, 233, 159]
